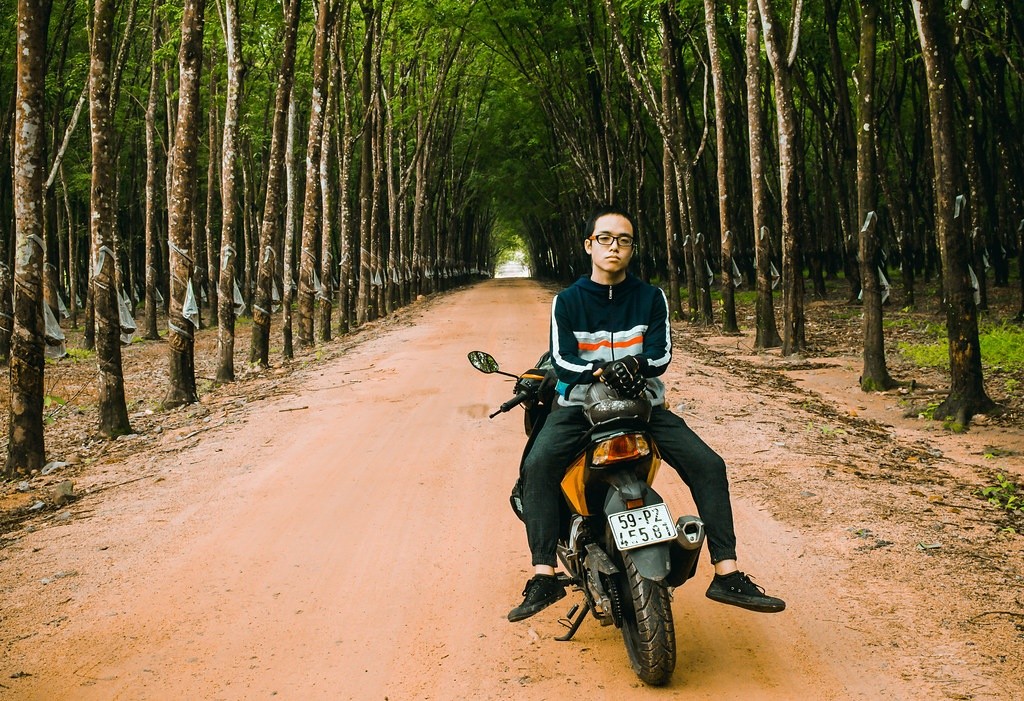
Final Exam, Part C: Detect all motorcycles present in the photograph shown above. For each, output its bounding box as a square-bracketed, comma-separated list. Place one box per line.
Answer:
[468, 349, 706, 688]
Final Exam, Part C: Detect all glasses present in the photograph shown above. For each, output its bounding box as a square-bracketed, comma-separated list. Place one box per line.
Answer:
[588, 234, 635, 247]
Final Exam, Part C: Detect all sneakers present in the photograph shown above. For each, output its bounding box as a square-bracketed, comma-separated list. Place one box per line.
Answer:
[508, 572, 567, 622]
[705, 570, 786, 613]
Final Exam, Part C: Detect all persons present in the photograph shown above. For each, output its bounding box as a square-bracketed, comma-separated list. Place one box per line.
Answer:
[509, 207, 788, 621]
